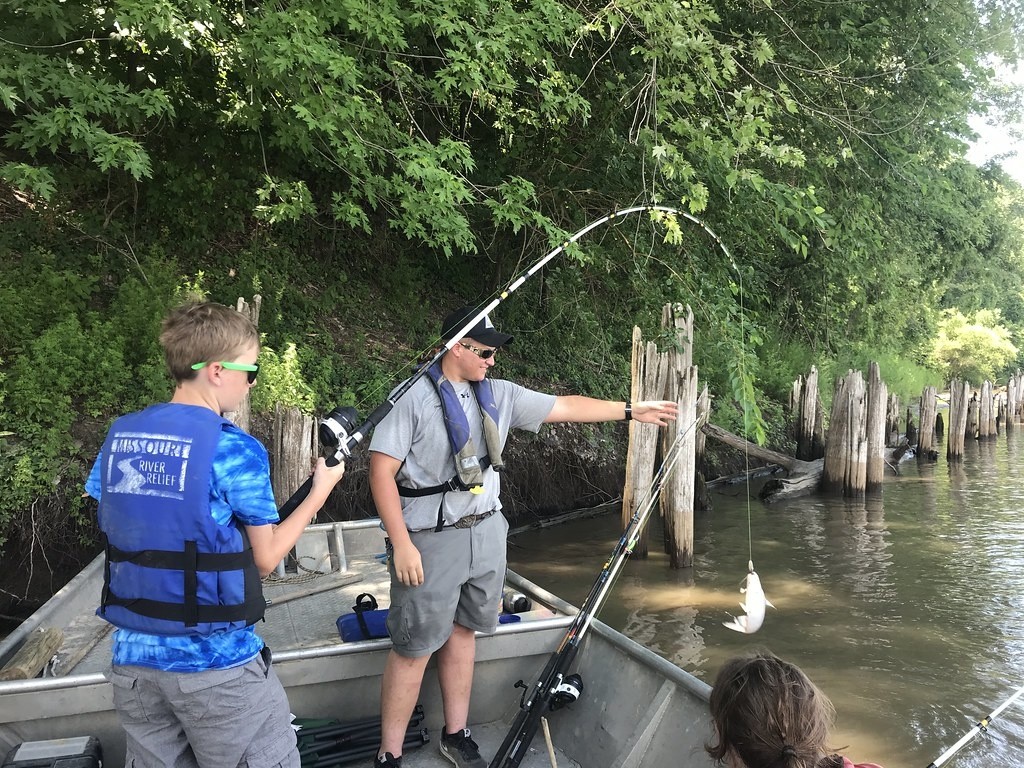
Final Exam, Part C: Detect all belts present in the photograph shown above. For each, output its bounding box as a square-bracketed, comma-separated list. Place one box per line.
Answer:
[443, 510, 494, 529]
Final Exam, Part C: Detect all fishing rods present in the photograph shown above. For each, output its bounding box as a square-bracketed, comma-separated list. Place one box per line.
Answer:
[924, 687, 1024, 768]
[279, 205, 752, 569]
[487, 412, 707, 768]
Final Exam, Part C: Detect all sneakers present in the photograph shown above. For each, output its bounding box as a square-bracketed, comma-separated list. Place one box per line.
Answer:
[440, 725, 487, 768]
[374, 748, 402, 768]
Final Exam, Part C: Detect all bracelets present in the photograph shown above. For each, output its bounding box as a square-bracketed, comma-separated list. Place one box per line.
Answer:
[625, 402, 632, 420]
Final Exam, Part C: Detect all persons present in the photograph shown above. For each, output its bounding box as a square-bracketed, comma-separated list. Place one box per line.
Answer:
[368, 309, 680, 768]
[705, 655, 883, 768]
[85, 303, 345, 768]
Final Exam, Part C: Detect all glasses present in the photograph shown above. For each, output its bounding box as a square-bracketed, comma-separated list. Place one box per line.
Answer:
[458, 340, 498, 360]
[191, 359, 260, 384]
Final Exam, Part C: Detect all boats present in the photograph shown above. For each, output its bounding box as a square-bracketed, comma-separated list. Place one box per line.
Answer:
[1, 517, 746, 768]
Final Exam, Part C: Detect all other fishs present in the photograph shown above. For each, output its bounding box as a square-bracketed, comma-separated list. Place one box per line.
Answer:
[718, 571, 778, 635]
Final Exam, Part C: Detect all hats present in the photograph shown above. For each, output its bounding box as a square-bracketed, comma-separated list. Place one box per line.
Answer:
[441, 308, 515, 347]
